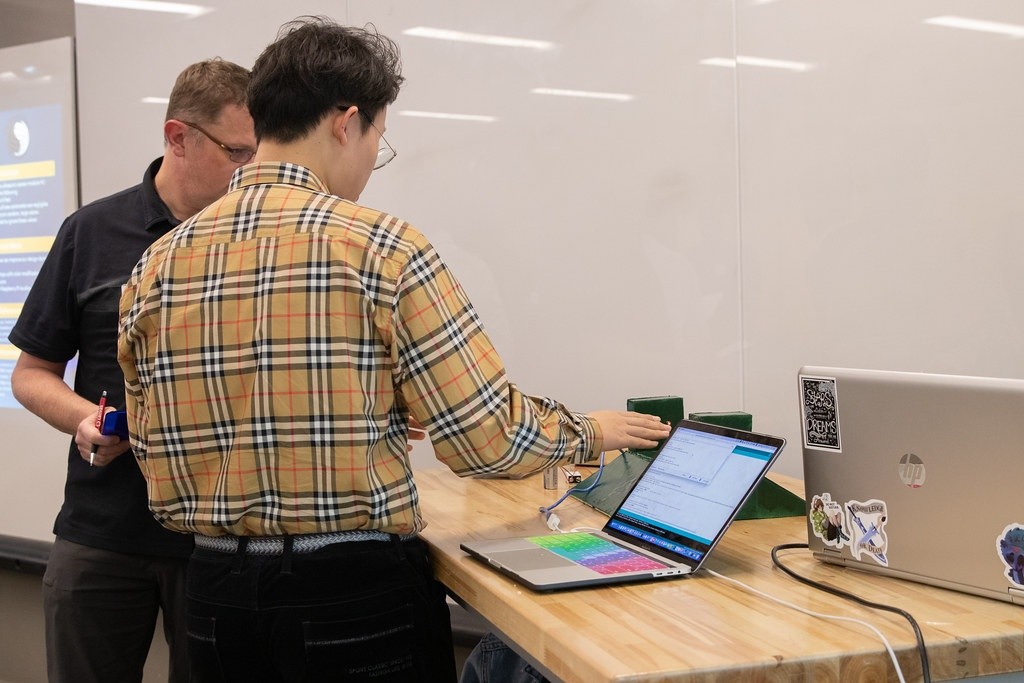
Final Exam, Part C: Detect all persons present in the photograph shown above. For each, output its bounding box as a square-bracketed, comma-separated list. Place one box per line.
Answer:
[114, 13, 675, 683]
[8, 58, 262, 682]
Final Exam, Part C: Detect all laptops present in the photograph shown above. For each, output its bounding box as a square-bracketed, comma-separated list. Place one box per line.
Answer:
[797, 365, 1024, 606]
[460, 419, 787, 593]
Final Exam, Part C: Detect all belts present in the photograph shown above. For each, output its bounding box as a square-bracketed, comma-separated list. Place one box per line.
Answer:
[194, 531, 417, 556]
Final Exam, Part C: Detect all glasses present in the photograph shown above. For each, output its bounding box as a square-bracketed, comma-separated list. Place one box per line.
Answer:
[336, 105, 397, 170]
[177, 118, 256, 164]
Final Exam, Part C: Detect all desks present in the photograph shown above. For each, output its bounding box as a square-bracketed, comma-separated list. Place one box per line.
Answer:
[410, 465, 1024, 683]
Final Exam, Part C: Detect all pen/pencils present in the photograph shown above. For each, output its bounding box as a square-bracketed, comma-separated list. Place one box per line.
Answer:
[89, 391, 107, 467]
